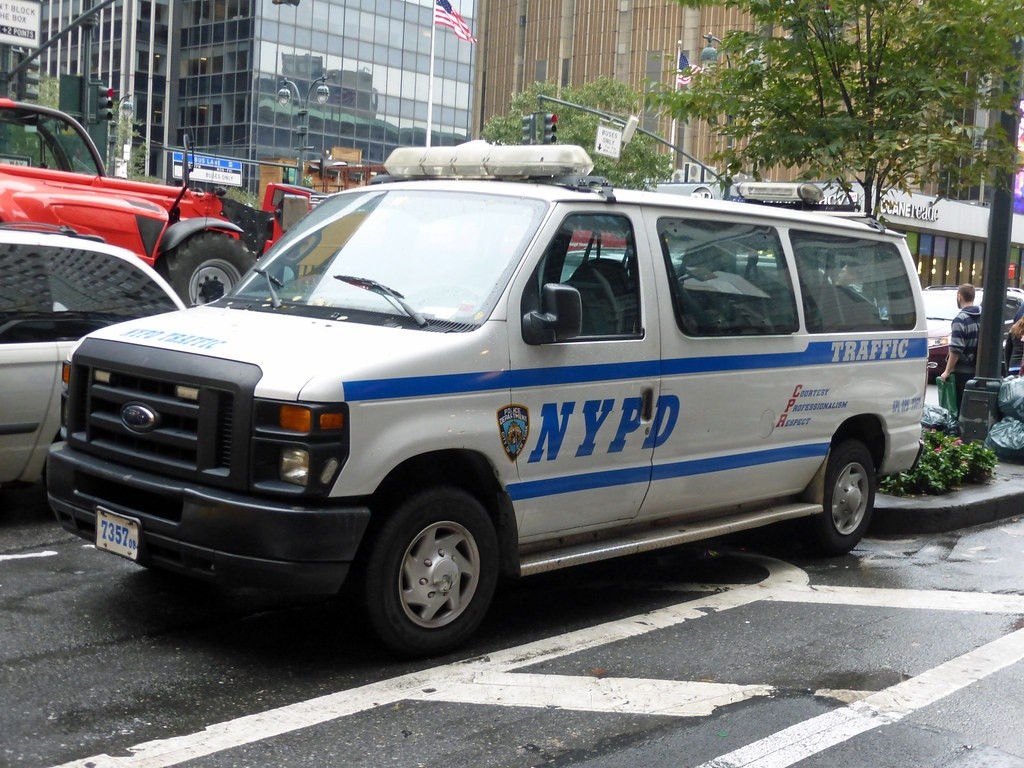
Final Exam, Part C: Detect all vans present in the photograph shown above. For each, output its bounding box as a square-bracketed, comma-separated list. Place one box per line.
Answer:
[46, 142, 929, 650]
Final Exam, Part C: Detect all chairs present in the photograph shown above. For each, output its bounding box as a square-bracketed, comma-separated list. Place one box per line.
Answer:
[557, 257, 881, 332]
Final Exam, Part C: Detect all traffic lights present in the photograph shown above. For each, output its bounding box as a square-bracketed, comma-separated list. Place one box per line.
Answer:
[522, 115, 536, 145]
[544, 112, 557, 145]
[97, 86, 113, 122]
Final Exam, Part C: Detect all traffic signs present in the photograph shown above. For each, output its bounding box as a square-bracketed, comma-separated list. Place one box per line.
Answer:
[593, 126, 623, 159]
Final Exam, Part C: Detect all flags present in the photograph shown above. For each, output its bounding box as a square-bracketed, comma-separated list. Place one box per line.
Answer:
[432, 0, 478, 43]
[676, 47, 701, 84]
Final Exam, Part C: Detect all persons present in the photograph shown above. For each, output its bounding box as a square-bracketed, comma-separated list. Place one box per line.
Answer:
[941, 283, 983, 413]
[837, 261, 870, 302]
[1004, 301, 1024, 378]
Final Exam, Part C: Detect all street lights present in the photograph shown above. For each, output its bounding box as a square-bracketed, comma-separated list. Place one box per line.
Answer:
[277, 73, 330, 186]
[698, 32, 734, 200]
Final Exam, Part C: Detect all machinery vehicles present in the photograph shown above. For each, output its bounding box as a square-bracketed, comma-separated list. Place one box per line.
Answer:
[0, 97, 328, 311]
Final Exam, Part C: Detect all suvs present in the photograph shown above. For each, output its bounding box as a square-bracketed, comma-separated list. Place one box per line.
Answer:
[559, 249, 882, 320]
[0, 226, 190, 488]
[920, 285, 1024, 380]
